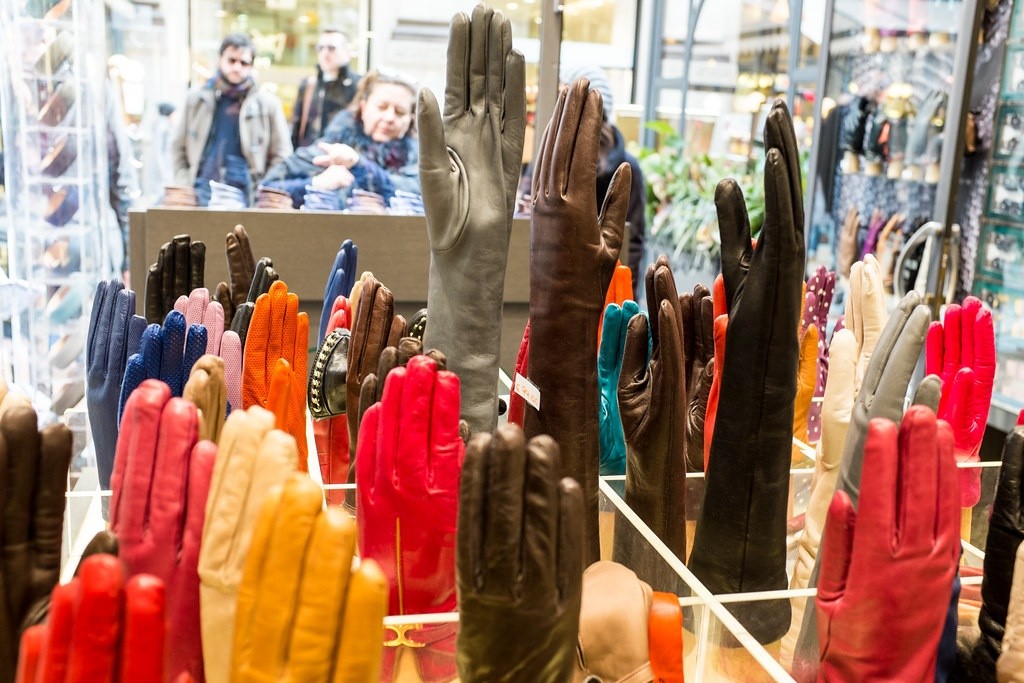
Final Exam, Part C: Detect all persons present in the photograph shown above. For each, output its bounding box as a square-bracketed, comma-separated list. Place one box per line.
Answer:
[816, 70, 886, 214]
[165, 34, 294, 209]
[291, 26, 367, 151]
[258, 69, 423, 200]
[517, 69, 646, 302]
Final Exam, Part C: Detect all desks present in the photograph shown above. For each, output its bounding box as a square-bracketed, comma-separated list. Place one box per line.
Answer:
[127, 205, 529, 378]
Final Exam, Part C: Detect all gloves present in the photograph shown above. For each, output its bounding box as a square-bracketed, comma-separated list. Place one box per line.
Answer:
[350, 355, 471, 683]
[526, 73, 628, 559]
[85, 276, 148, 523]
[920, 294, 997, 509]
[412, 1, 529, 434]
[678, 100, 806, 649]
[0, 401, 119, 683]
[15, 553, 197, 683]
[813, 404, 963, 683]
[507, 198, 897, 603]
[110, 223, 452, 513]
[793, 290, 945, 683]
[453, 422, 586, 682]
[106, 378, 219, 678]
[198, 406, 427, 683]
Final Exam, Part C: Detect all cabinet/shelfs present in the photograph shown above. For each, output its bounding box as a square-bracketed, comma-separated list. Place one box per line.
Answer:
[802, 0, 1024, 433]
[0, 0, 121, 542]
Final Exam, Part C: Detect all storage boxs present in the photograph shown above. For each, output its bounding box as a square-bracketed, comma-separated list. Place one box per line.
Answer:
[52, 363, 1024, 683]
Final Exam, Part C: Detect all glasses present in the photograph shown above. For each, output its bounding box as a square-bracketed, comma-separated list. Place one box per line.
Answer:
[315, 44, 339, 54]
[224, 55, 252, 68]
